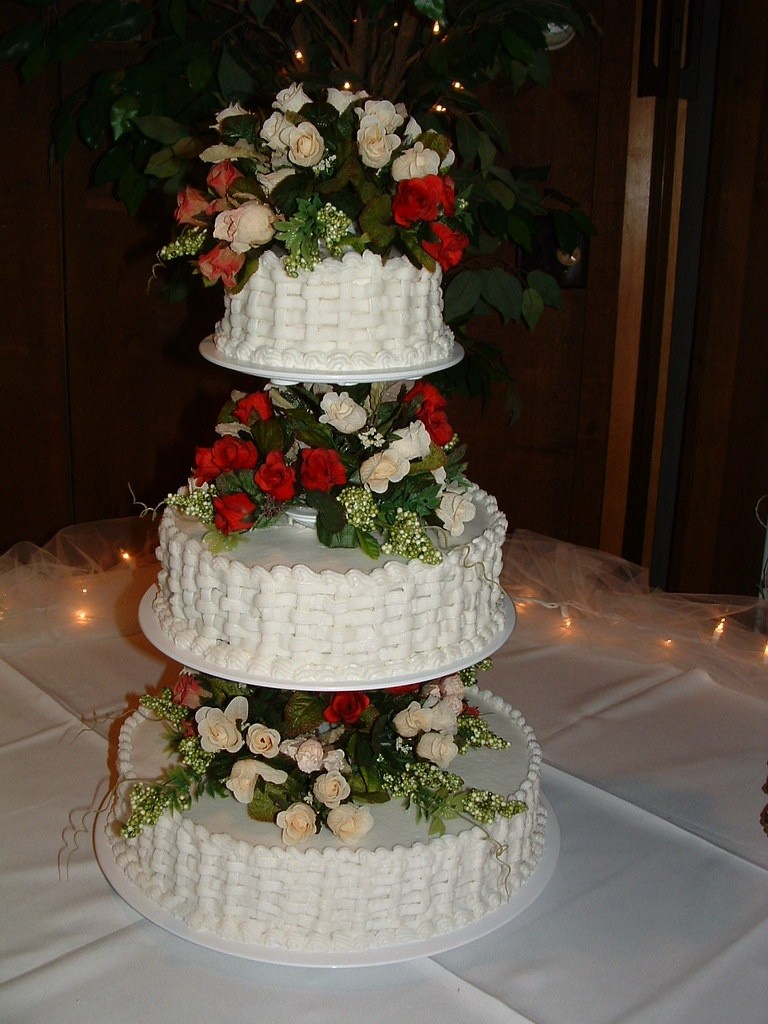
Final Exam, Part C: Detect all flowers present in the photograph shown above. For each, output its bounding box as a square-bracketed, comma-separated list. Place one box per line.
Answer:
[167, 382, 475, 566]
[149, 75, 483, 296]
[119, 658, 529, 841]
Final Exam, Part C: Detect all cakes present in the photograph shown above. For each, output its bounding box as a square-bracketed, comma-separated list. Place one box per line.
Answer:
[104, 81, 544, 955]
